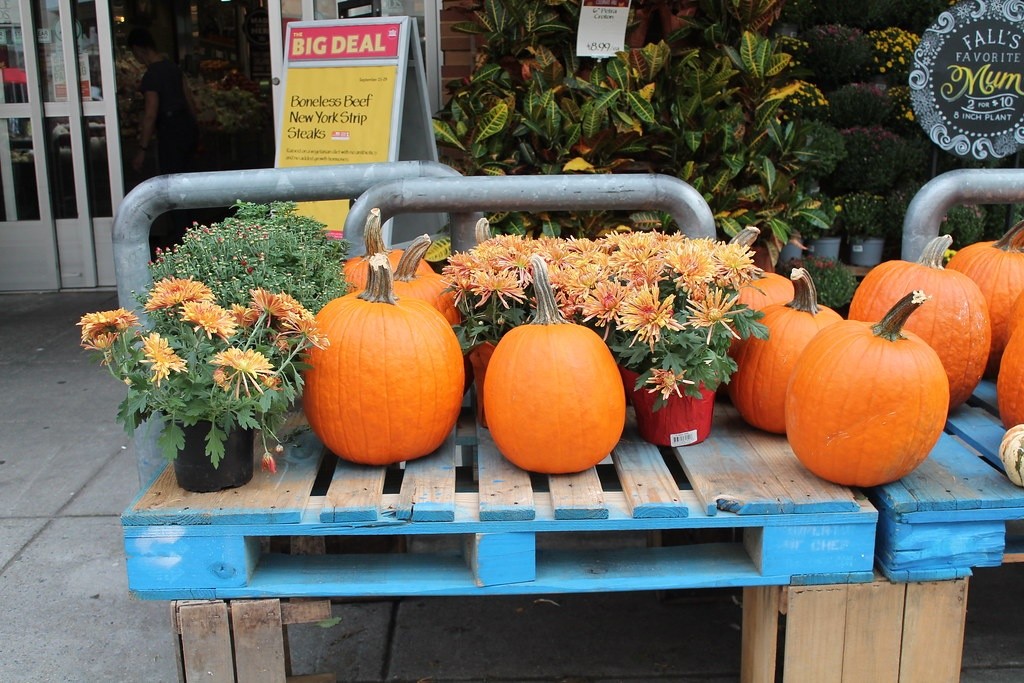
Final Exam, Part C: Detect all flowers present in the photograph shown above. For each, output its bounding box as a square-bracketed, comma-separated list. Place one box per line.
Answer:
[73, 279, 331, 472]
[440, 231, 769, 414]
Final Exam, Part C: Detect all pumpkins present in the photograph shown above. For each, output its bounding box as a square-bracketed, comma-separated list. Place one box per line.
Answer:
[298, 208, 465, 464]
[484, 254, 626, 475]
[711, 218, 1024, 489]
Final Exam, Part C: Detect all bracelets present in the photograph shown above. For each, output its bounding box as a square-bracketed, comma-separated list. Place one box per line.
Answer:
[138, 144, 148, 152]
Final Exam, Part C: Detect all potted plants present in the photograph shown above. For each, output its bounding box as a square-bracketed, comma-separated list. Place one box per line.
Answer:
[811, 194, 846, 261]
[838, 191, 886, 267]
[718, 40, 821, 273]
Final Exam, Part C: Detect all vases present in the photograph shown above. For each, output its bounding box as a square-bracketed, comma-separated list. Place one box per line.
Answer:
[163, 411, 254, 492]
[781, 238, 802, 261]
[469, 343, 498, 428]
[622, 368, 718, 448]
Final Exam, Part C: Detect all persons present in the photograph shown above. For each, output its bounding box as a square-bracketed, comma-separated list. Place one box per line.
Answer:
[127, 28, 200, 173]
